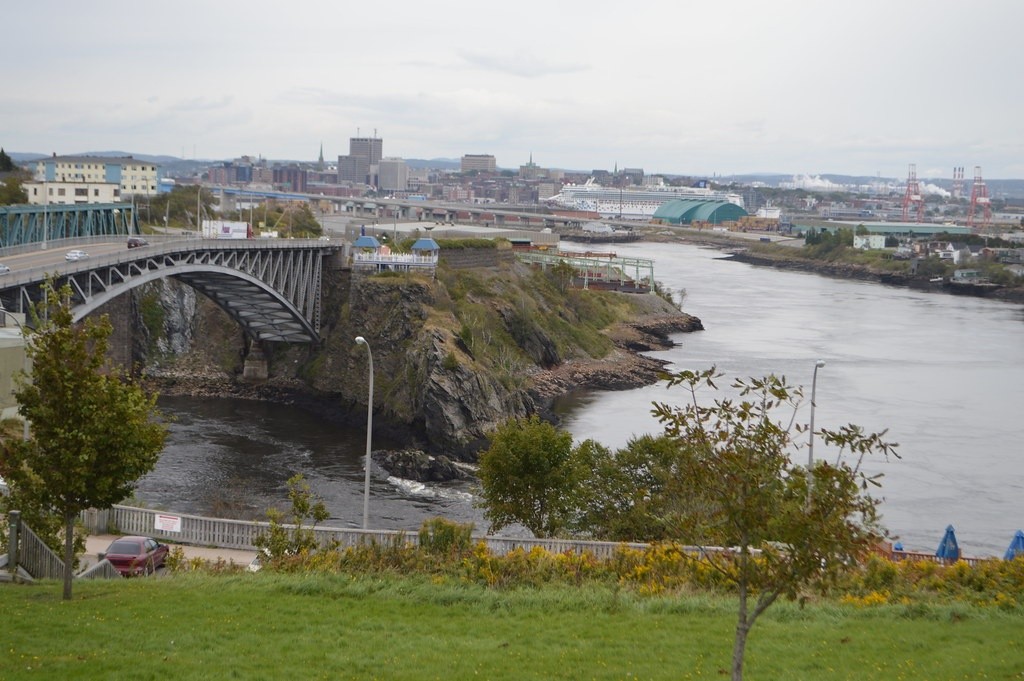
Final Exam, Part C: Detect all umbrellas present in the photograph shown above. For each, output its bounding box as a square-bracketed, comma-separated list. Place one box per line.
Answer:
[891, 541, 906, 562]
[1003, 529, 1024, 561]
[935, 524, 958, 563]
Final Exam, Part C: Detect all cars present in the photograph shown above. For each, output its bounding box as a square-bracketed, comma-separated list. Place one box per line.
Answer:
[101, 534, 169, 578]
[65, 249, 91, 261]
[318, 236, 329, 241]
[245, 546, 290, 573]
[0, 262, 11, 274]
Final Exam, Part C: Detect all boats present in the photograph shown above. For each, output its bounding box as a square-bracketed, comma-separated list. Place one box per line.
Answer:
[949, 267, 1003, 298]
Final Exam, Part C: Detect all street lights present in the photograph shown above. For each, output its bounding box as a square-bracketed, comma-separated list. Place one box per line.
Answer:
[806, 358, 826, 510]
[352, 335, 375, 529]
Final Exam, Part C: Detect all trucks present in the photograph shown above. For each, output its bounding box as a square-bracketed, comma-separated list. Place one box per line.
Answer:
[202, 220, 255, 241]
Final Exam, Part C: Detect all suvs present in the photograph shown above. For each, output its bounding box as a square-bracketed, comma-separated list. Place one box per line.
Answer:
[128, 236, 151, 249]
